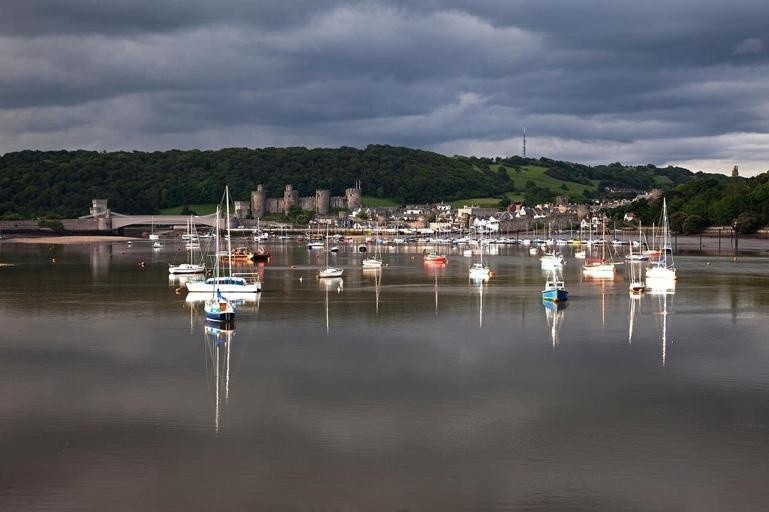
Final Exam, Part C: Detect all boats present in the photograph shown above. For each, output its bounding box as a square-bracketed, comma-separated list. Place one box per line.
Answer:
[203, 288, 235, 325]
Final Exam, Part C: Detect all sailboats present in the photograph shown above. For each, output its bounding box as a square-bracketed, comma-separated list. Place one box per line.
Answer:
[179, 293, 264, 329]
[543, 305, 566, 349]
[200, 320, 238, 433]
[648, 286, 675, 368]
[541, 249, 569, 306]
[625, 293, 643, 345]
